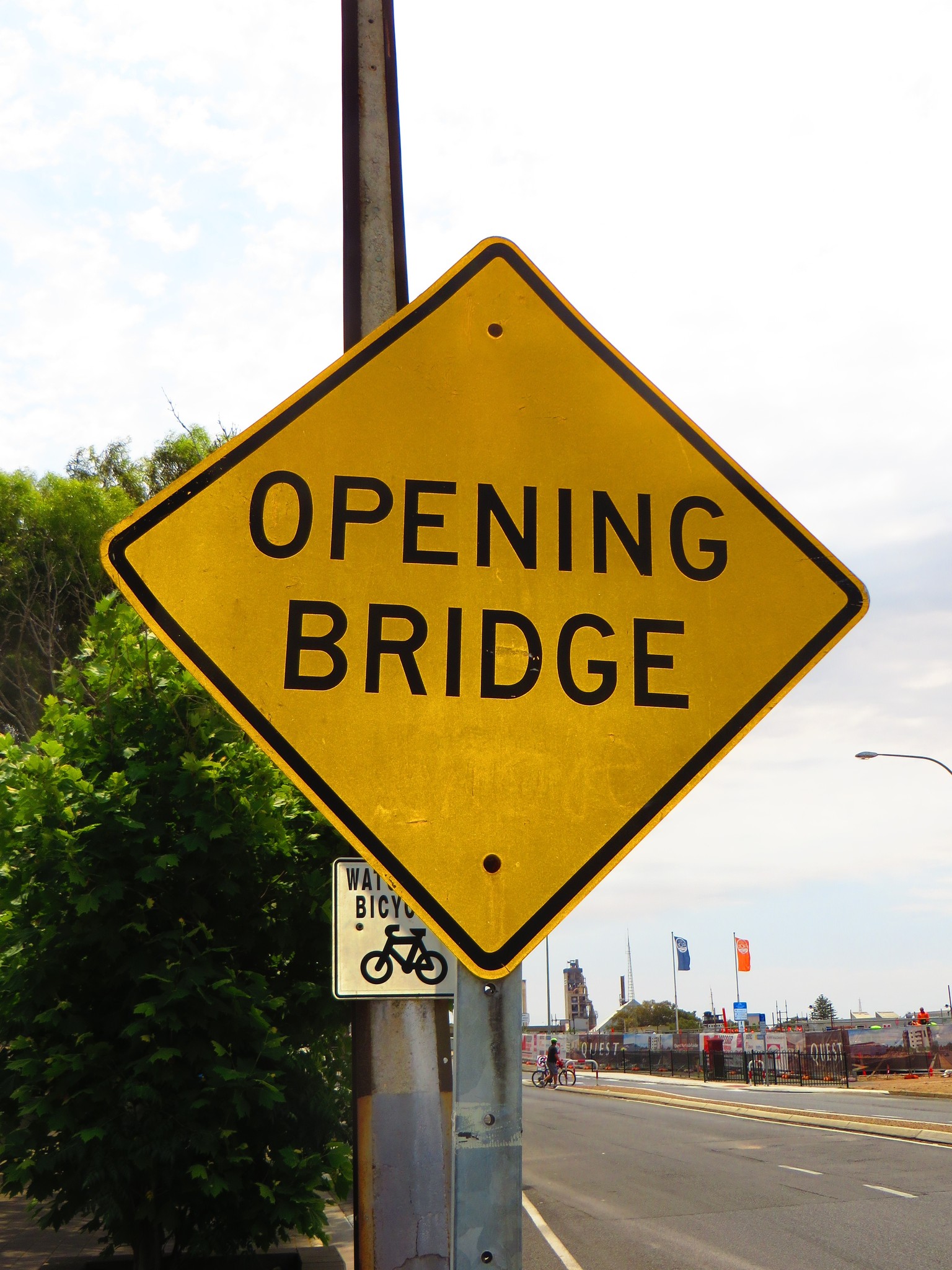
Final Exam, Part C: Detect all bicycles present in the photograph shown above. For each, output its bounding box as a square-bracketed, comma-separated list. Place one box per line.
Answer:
[531, 1059, 576, 1088]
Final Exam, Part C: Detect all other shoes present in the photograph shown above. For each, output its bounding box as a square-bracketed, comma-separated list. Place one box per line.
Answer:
[540, 1080, 545, 1088]
[554, 1084, 560, 1088]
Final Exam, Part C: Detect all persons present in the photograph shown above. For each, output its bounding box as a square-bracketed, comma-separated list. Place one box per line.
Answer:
[540, 1038, 562, 1088]
[911, 1008, 929, 1025]
[611, 1027, 615, 1034]
[944, 1005, 951, 1016]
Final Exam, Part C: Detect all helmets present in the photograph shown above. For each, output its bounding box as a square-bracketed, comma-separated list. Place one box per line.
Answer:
[912, 1015, 916, 1018]
[551, 1038, 558, 1042]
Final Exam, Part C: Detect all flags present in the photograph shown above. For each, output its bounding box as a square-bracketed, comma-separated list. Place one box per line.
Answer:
[735, 937, 750, 971]
[674, 936, 690, 971]
